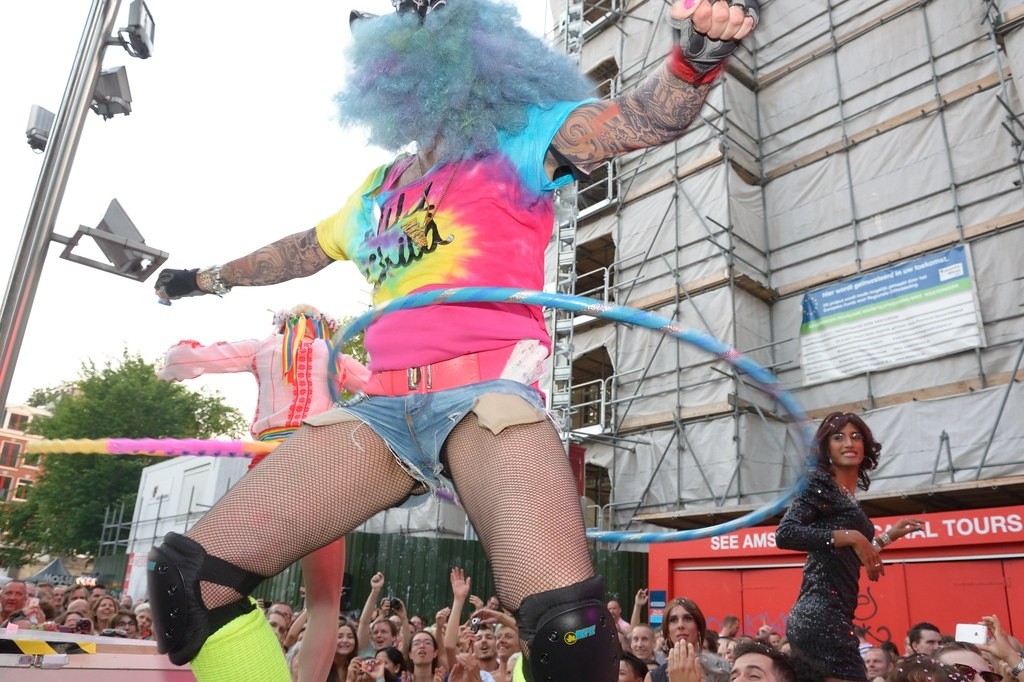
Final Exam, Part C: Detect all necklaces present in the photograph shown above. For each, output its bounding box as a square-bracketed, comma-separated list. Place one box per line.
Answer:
[401, 140, 464, 248]
[838, 482, 858, 507]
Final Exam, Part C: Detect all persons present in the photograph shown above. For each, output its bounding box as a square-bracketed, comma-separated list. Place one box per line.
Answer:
[0, 565, 1024, 682]
[775, 412, 926, 682]
[147, 0, 763, 682]
[159, 303, 373, 682]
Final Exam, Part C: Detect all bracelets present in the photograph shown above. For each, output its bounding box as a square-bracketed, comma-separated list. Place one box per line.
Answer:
[1012, 659, 1024, 676]
[668, 42, 720, 86]
[871, 540, 882, 552]
[878, 532, 891, 545]
[200, 266, 231, 299]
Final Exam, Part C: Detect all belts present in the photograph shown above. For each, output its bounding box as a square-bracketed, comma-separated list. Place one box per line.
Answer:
[364, 343, 539, 397]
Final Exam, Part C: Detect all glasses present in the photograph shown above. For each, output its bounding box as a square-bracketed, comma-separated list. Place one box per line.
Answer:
[831, 432, 863, 443]
[951, 663, 1003, 682]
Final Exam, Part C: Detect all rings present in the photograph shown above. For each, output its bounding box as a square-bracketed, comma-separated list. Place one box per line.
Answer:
[875, 563, 879, 567]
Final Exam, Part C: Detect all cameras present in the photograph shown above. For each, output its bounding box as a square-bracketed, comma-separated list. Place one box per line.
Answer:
[358, 658, 374, 675]
[470, 617, 481, 635]
[388, 599, 400, 609]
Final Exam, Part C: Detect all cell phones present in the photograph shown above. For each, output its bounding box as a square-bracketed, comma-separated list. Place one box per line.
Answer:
[955, 624, 988, 645]
[57, 625, 77, 633]
[29, 598, 40, 615]
